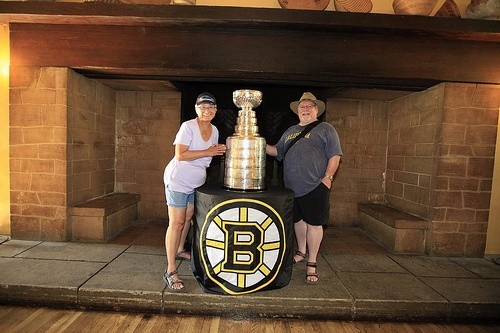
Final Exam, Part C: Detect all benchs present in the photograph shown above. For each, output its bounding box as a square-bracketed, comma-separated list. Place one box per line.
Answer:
[357, 204, 428, 255]
[70, 193, 141, 243]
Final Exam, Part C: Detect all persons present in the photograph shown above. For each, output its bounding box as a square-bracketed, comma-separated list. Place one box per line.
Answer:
[162, 94, 227, 290]
[265, 92, 343, 285]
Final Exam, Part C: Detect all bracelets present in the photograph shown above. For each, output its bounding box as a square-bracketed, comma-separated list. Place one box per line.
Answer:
[326, 175, 333, 182]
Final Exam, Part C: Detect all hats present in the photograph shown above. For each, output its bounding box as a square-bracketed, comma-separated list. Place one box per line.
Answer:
[196, 92, 216, 104]
[290, 92, 325, 117]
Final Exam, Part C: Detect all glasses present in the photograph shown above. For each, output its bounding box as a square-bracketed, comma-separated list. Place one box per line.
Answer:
[197, 104, 215, 110]
[296, 104, 316, 109]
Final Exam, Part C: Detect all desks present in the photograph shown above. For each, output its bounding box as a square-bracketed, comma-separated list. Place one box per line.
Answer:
[193, 189, 292, 295]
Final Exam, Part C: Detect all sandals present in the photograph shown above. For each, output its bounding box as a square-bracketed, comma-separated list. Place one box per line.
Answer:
[176, 249, 190, 260]
[305, 262, 319, 284]
[163, 269, 185, 291]
[292, 250, 307, 264]
